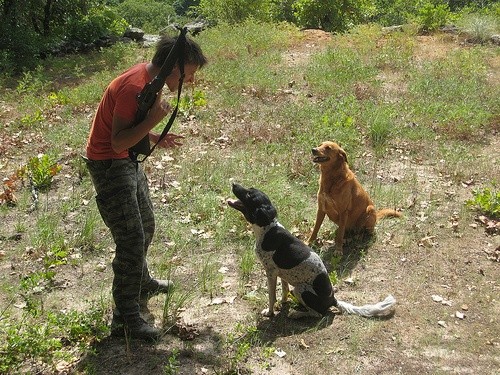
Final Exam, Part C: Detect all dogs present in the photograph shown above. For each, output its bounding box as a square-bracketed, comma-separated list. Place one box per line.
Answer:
[307, 141, 400, 257]
[227, 183, 396, 318]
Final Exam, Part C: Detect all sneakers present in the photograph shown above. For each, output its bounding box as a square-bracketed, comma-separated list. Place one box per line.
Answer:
[141, 279, 175, 295]
[110, 312, 162, 341]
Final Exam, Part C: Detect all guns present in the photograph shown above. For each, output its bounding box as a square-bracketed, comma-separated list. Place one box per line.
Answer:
[129, 26, 189, 156]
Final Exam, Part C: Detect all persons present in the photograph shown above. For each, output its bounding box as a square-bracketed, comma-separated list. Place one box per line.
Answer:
[85, 34, 209, 341]
